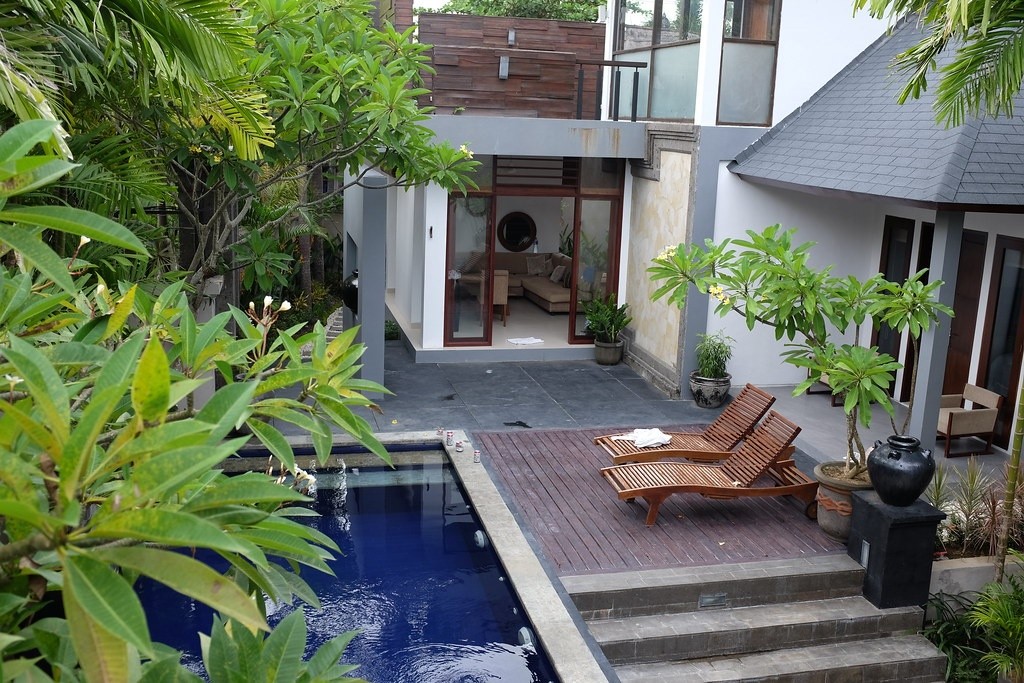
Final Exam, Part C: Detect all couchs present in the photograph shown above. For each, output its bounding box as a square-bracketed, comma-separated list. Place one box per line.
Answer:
[454, 250, 604, 317]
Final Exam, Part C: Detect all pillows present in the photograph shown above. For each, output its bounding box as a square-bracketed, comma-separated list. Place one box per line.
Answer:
[526, 254, 576, 289]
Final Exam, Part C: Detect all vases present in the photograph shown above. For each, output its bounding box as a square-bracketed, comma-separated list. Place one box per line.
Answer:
[867, 434, 935, 507]
[343, 270, 358, 315]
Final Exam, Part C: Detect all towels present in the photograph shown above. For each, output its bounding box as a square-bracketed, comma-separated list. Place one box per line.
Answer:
[610, 428, 673, 449]
[505, 337, 545, 346]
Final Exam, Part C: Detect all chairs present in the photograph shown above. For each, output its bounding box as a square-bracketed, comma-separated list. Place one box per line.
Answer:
[477, 269, 509, 327]
[937, 382, 1004, 459]
[592, 382, 821, 528]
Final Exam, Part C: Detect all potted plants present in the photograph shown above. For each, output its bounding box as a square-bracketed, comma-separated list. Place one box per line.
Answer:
[580, 294, 634, 366]
[652, 229, 957, 544]
[689, 327, 736, 409]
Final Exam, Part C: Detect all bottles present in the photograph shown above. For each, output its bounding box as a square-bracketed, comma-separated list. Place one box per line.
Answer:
[533, 236, 539, 255]
[867, 435, 936, 507]
